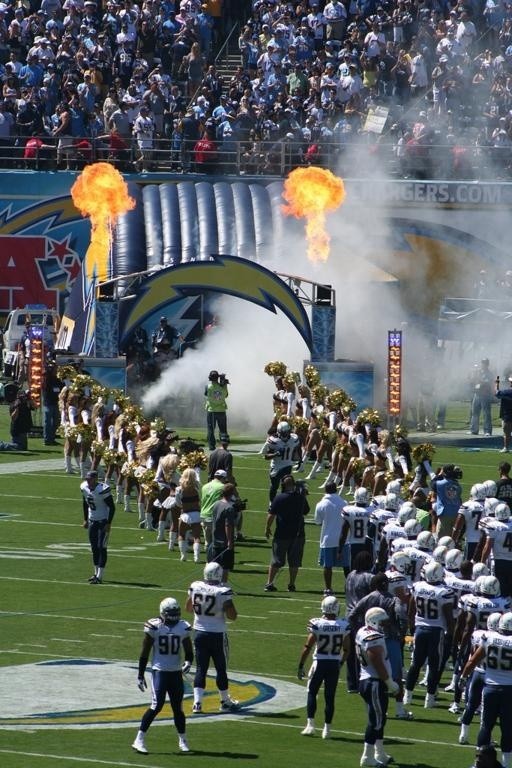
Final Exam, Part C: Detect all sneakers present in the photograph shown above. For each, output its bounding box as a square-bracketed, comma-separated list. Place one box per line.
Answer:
[425, 700, 440, 708]
[402, 696, 412, 705]
[444, 684, 469, 744]
[323, 588, 331, 596]
[178, 742, 189, 752]
[360, 752, 392, 767]
[192, 703, 201, 713]
[264, 585, 277, 592]
[322, 732, 329, 739]
[397, 710, 413, 719]
[300, 727, 315, 735]
[132, 740, 148, 753]
[220, 699, 238, 713]
[288, 584, 295, 592]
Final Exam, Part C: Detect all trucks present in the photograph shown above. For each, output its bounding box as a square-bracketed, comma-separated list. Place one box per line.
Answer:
[2, 306, 62, 375]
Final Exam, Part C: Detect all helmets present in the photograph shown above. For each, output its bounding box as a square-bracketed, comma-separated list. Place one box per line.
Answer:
[459, 560, 512, 632]
[159, 597, 181, 626]
[354, 480, 420, 537]
[366, 607, 388, 631]
[204, 562, 223, 583]
[471, 480, 511, 522]
[390, 530, 461, 584]
[320, 596, 340, 618]
[278, 422, 292, 440]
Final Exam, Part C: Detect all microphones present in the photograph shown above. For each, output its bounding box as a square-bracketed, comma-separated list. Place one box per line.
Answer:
[496, 376, 500, 383]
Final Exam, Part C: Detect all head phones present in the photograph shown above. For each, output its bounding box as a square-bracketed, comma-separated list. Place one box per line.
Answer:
[281, 475, 295, 487]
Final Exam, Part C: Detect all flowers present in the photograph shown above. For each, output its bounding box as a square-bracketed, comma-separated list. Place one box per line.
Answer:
[264, 361, 436, 499]
[55, 366, 208, 499]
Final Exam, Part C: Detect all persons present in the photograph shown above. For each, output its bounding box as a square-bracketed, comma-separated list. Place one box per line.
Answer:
[0, 390, 32, 451]
[1, 0, 512, 180]
[186, 562, 241, 715]
[262, 357, 512, 767]
[132, 597, 193, 752]
[46, 316, 245, 585]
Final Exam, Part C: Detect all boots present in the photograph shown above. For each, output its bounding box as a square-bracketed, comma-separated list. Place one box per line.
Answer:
[297, 454, 348, 495]
[116, 485, 155, 531]
[157, 521, 200, 563]
[65, 454, 88, 479]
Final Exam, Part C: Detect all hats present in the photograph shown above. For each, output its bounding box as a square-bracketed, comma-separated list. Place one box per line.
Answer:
[86, 470, 98, 479]
[214, 469, 227, 476]
